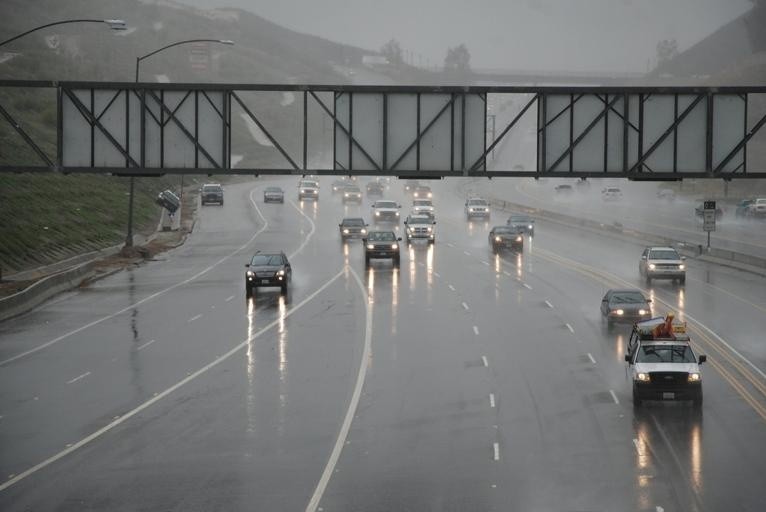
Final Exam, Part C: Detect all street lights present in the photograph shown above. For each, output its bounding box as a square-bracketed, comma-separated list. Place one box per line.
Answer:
[0, 19, 128, 46]
[126, 39, 235, 246]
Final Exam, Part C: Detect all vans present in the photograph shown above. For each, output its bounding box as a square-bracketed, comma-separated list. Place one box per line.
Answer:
[639, 245, 686, 284]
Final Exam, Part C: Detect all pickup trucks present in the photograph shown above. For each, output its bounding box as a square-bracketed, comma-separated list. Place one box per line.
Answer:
[624, 317, 706, 407]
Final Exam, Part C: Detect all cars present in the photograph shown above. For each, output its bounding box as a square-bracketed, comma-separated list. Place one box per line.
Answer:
[694, 204, 724, 220]
[157, 173, 436, 295]
[735, 198, 766, 218]
[488, 225, 524, 254]
[600, 186, 625, 201]
[600, 288, 652, 328]
[507, 215, 535, 236]
[657, 189, 676, 202]
[553, 179, 591, 196]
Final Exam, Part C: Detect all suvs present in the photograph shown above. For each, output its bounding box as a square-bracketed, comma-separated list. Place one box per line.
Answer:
[465, 198, 491, 221]
